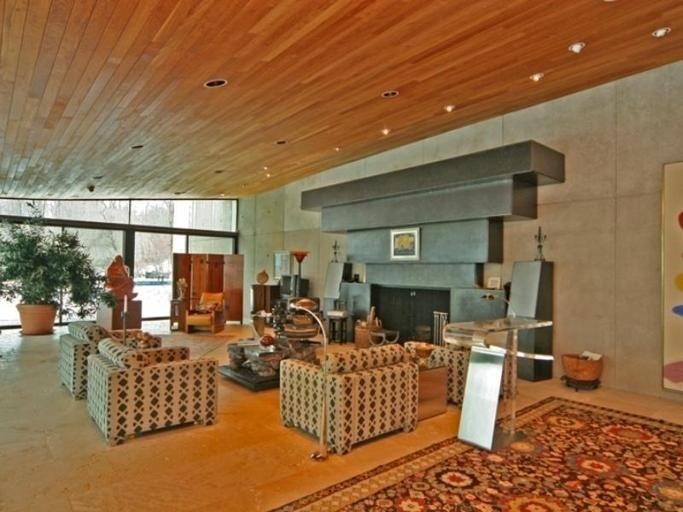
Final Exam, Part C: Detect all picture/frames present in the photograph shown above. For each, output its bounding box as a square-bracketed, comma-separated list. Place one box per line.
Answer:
[271, 248, 291, 280]
[388, 227, 421, 262]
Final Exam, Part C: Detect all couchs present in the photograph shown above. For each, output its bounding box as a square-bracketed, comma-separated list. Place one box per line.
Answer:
[85, 337, 217, 447]
[58, 319, 161, 402]
[402, 332, 469, 412]
[187, 291, 229, 331]
[280, 340, 419, 456]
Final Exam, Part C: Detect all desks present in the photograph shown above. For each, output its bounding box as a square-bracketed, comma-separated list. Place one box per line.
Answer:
[170, 299, 188, 332]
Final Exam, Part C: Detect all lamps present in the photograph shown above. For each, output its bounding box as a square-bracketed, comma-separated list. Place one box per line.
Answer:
[289, 250, 309, 297]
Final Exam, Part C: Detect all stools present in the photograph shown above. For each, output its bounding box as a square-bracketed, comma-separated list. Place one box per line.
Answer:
[325, 310, 348, 345]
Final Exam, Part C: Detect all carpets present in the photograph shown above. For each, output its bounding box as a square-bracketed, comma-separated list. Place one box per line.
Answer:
[268, 394, 682, 512]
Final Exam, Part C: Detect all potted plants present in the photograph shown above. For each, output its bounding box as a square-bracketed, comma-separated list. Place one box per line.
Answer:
[176, 277, 187, 300]
[0, 207, 117, 336]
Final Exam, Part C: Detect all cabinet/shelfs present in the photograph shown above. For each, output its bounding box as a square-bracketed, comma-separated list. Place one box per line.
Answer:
[96, 299, 142, 330]
[251, 283, 278, 314]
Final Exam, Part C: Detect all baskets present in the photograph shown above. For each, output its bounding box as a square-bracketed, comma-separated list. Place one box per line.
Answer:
[354, 304, 381, 347]
